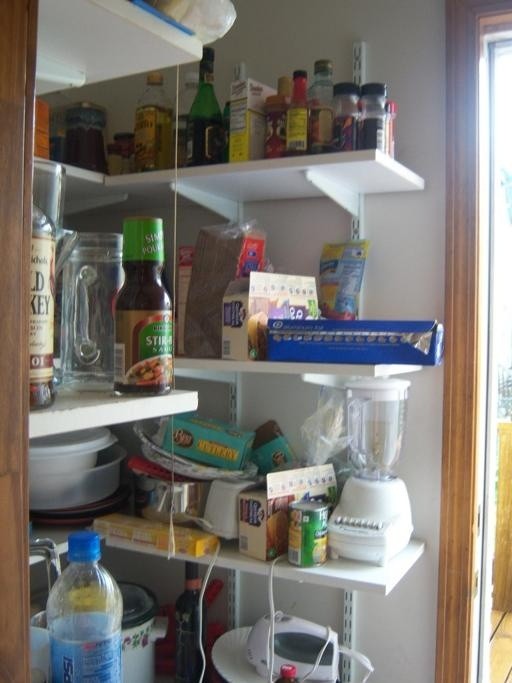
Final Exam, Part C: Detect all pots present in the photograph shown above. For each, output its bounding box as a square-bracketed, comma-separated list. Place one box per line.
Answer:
[132, 476, 209, 523]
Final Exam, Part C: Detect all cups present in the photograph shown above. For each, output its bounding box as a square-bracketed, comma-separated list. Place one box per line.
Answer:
[60, 231, 123, 390]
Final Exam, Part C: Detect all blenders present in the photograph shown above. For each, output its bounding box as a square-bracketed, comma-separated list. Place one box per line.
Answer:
[326, 376, 414, 563]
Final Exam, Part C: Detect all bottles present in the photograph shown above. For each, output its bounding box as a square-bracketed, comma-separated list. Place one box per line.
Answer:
[265, 59, 397, 159]
[113, 216, 174, 397]
[28, 196, 56, 409]
[112, 45, 230, 173]
[45, 530, 123, 682]
[174, 560, 206, 682]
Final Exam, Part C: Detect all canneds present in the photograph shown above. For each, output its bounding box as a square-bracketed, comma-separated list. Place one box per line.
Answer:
[289, 501, 329, 566]
[107, 133, 135, 174]
